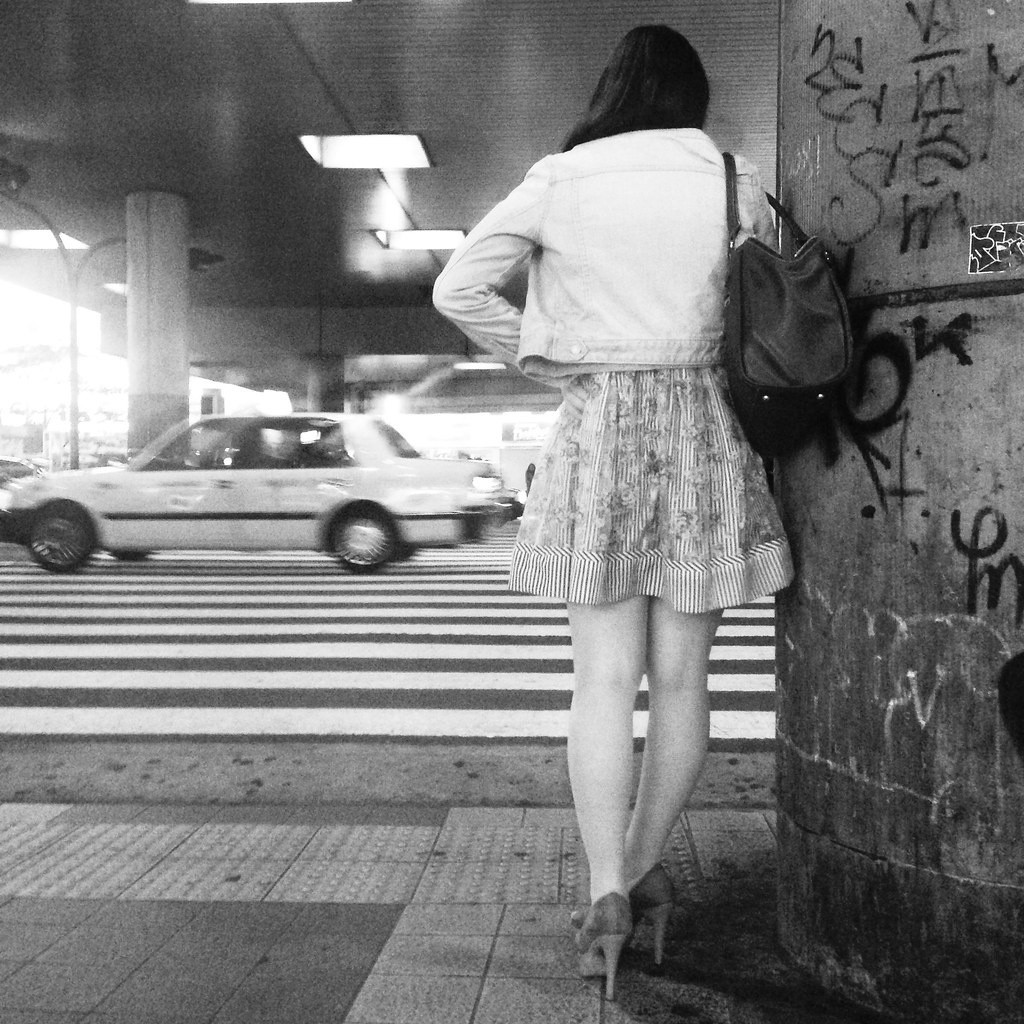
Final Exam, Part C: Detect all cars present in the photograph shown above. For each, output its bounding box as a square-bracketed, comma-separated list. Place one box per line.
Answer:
[1, 414, 521, 573]
[0, 448, 238, 542]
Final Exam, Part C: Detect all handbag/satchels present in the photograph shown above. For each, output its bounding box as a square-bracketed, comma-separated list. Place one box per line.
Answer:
[723, 152, 854, 458]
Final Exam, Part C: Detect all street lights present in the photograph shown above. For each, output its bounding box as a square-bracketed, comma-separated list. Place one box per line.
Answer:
[0, 159, 221, 470]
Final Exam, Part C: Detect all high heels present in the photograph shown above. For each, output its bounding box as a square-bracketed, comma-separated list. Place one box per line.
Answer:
[575, 892, 633, 1000]
[571, 863, 673, 967]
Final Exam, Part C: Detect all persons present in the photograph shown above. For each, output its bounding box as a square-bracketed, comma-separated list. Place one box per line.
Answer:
[526, 464, 535, 495]
[433, 23, 795, 997]
